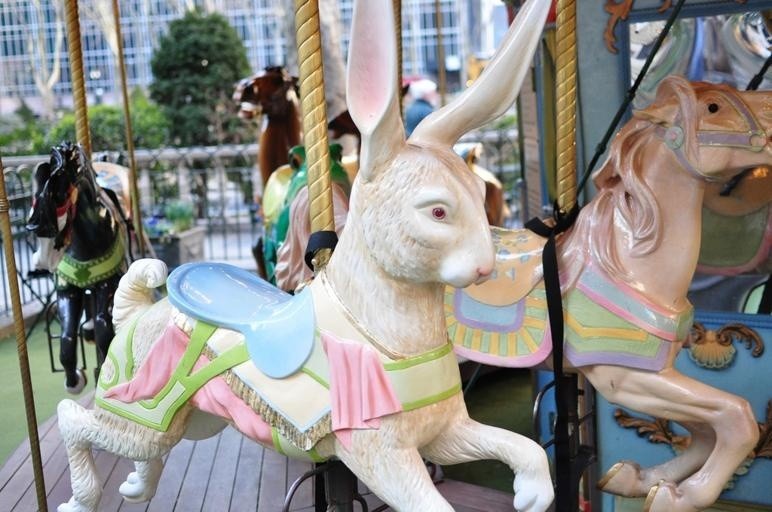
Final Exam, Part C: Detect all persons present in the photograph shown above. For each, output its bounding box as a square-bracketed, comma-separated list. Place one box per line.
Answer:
[405, 79, 440, 138]
